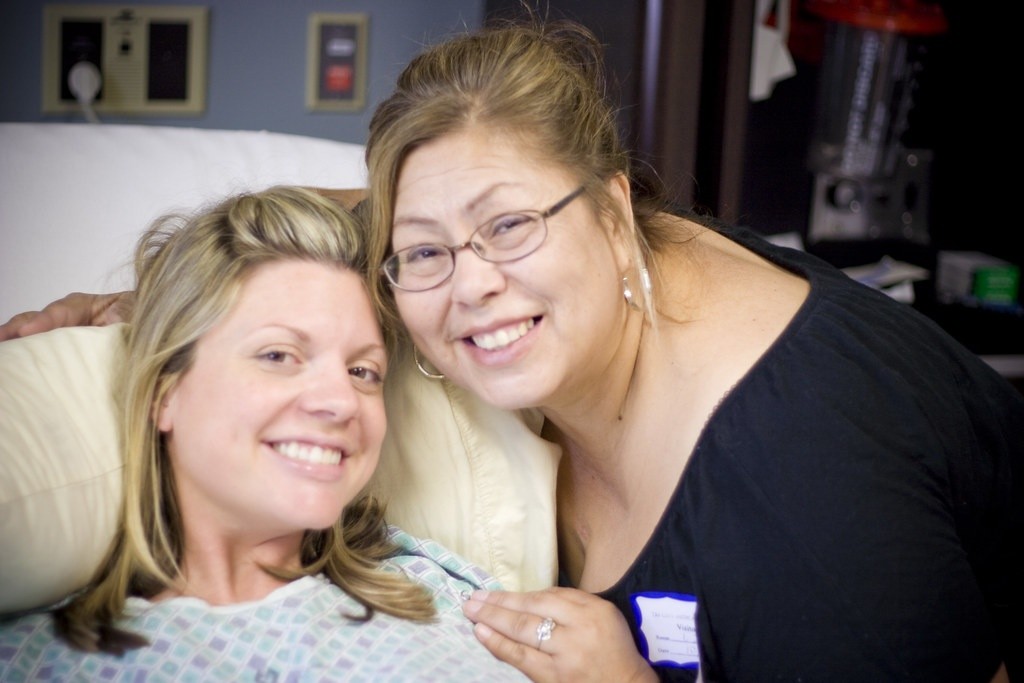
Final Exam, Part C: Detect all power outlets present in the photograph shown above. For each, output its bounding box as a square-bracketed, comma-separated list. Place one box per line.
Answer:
[43, 4, 208, 114]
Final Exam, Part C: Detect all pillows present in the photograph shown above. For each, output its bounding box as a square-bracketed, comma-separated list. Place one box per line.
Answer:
[1, 324, 567, 594]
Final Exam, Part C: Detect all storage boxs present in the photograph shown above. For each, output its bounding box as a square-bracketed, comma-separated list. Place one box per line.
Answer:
[937, 250, 1018, 304]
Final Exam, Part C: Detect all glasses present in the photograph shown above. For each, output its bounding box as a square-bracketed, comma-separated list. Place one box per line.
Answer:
[378, 186, 587, 292]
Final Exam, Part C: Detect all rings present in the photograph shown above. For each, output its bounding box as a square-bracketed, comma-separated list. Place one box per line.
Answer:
[535, 616, 556, 652]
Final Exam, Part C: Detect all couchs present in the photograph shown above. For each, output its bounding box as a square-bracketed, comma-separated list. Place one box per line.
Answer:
[0, 119, 370, 323]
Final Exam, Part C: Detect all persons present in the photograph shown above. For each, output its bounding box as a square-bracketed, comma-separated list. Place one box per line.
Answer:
[0, 0, 1024, 683]
